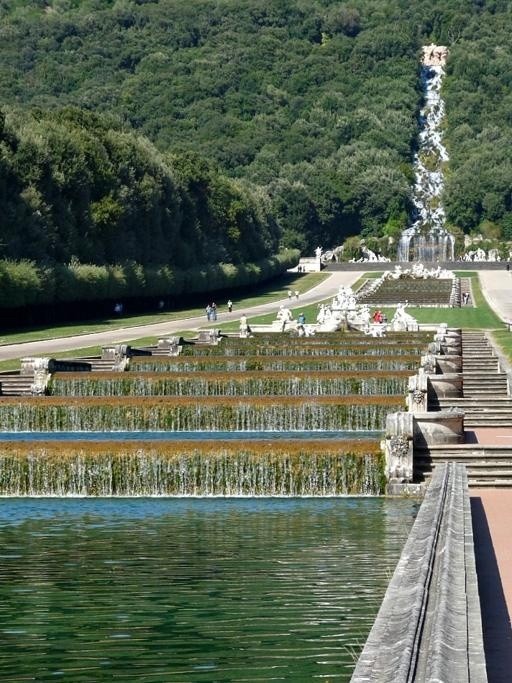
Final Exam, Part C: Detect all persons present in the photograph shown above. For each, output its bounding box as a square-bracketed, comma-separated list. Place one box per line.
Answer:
[239, 315, 246, 331]
[372, 308, 389, 325]
[276, 289, 306, 324]
[463, 293, 470, 305]
[206, 298, 233, 324]
[298, 266, 308, 274]
